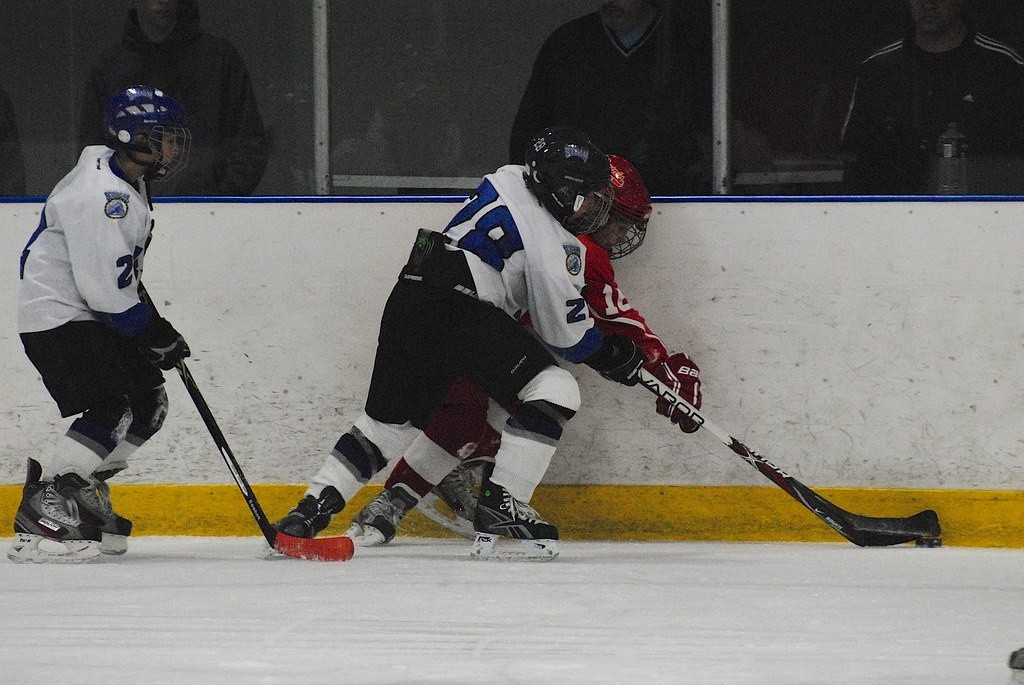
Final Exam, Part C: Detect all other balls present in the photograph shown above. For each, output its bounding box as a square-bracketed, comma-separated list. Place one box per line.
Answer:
[913, 536, 942, 548]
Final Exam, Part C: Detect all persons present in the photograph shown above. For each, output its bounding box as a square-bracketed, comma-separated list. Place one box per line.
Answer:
[7, 85, 190, 562]
[840, 0, 1024, 195]
[270, 132, 702, 563]
[510, 0, 712, 195]
[76, 0, 269, 198]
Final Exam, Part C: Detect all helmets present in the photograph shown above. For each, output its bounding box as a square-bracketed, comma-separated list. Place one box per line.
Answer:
[101, 85, 190, 182]
[523, 126, 610, 235]
[593, 154, 651, 258]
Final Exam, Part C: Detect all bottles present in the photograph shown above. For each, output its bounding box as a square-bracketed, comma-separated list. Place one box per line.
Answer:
[938, 122, 967, 195]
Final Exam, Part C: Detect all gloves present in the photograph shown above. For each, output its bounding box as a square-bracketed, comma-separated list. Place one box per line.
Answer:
[652, 353, 702, 434]
[135, 317, 190, 370]
[594, 334, 649, 386]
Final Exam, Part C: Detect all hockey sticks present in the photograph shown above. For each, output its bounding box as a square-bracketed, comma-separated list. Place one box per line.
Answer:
[137, 280, 356, 563]
[632, 365, 941, 549]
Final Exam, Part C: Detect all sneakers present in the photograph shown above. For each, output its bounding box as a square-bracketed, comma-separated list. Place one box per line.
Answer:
[417, 458, 484, 540]
[257, 486, 345, 557]
[467, 477, 559, 562]
[72, 461, 132, 554]
[341, 483, 421, 547]
[6, 456, 107, 564]
